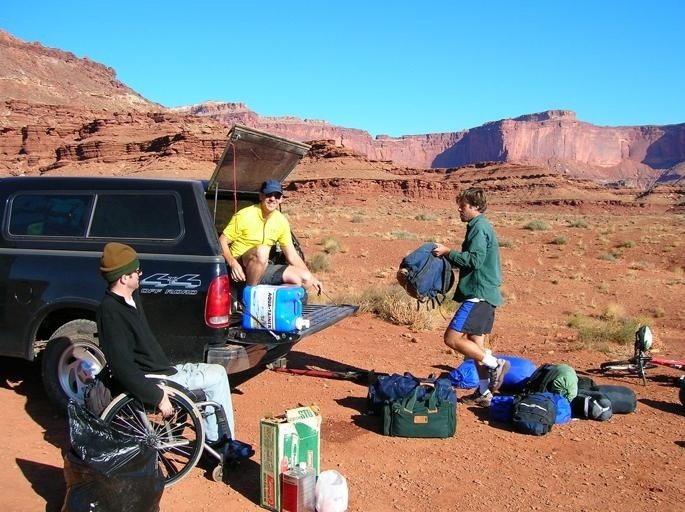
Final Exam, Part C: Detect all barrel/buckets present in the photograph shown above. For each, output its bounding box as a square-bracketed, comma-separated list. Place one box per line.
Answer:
[242, 284, 311, 334]
[281, 462, 316, 512]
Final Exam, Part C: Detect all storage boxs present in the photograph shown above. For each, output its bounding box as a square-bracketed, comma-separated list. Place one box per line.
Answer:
[259, 403, 322, 511]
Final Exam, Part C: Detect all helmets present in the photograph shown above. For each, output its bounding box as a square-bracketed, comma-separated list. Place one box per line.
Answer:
[639, 325, 653, 353]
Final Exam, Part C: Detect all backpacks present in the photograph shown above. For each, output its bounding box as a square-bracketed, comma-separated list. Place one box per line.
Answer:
[396, 243, 455, 312]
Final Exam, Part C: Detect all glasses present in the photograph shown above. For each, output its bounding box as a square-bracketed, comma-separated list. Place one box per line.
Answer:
[264, 192, 282, 198]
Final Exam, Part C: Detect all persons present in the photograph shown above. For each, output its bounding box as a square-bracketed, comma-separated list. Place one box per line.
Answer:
[95, 240, 256, 463]
[430, 185, 512, 409]
[219, 179, 323, 317]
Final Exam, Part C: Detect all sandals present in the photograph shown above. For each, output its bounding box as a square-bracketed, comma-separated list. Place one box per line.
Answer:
[206, 440, 254, 466]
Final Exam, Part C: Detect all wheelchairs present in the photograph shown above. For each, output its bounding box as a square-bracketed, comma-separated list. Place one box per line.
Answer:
[81, 332, 256, 485]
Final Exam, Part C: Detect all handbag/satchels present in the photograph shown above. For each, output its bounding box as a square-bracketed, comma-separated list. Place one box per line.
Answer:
[366, 363, 637, 439]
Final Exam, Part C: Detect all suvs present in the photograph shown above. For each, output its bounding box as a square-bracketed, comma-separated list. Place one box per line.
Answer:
[0, 120, 363, 419]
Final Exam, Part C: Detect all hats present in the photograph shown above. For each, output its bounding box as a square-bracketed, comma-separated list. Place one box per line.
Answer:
[260, 180, 283, 196]
[99, 242, 140, 282]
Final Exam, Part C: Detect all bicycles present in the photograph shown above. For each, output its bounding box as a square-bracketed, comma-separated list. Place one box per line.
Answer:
[600, 332, 685, 387]
